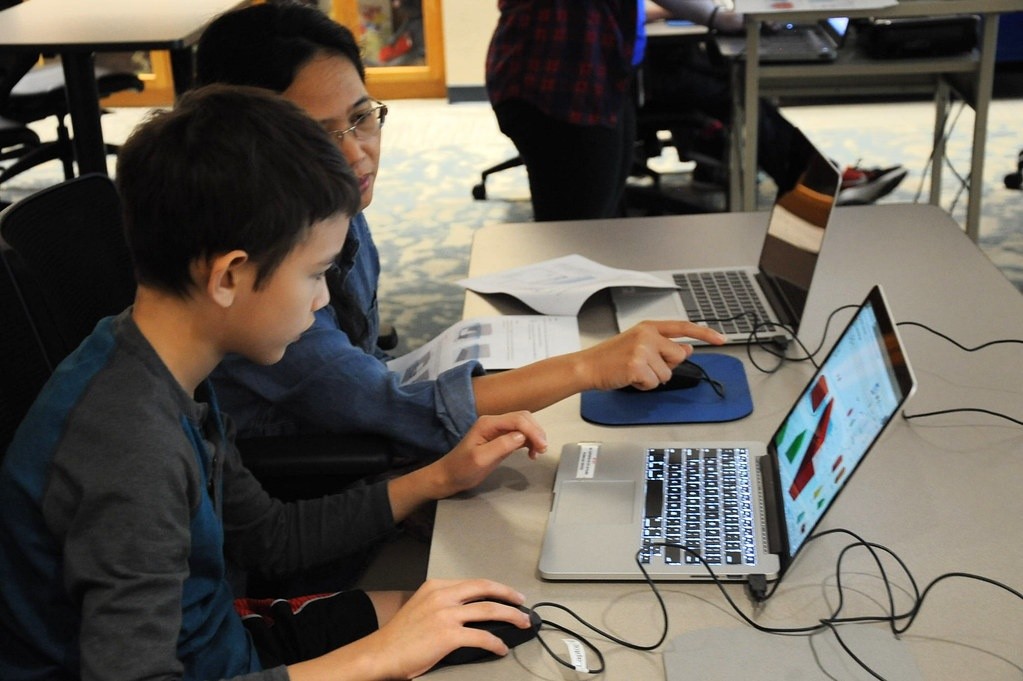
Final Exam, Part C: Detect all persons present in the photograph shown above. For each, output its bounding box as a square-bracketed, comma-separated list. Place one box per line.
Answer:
[0, 84, 548, 681]
[195, 0, 727, 594]
[485, 0, 910, 222]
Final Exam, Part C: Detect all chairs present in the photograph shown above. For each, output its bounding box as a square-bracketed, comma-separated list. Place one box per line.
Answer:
[0, 176, 393, 472]
[473, 25, 730, 216]
[0, 44, 144, 184]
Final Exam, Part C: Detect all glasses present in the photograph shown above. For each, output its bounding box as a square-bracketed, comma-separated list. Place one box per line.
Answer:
[325, 96, 388, 146]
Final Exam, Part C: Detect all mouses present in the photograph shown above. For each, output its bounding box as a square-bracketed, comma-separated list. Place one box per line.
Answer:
[426, 595, 542, 670]
[613, 359, 703, 393]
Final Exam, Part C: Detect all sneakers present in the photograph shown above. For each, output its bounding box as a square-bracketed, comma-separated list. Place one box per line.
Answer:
[691, 162, 761, 187]
[835, 158, 907, 206]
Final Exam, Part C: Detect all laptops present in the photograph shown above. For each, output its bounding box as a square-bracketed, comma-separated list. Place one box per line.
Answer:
[539, 284, 917, 582]
[610, 125, 843, 349]
[716, 16, 851, 64]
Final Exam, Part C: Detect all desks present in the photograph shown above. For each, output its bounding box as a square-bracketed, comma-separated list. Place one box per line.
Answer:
[412, 204, 1023, 681]
[730, 0, 1023, 243]
[0, 0, 249, 176]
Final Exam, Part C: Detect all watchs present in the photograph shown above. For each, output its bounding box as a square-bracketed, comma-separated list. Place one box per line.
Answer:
[709, 6, 721, 36]
